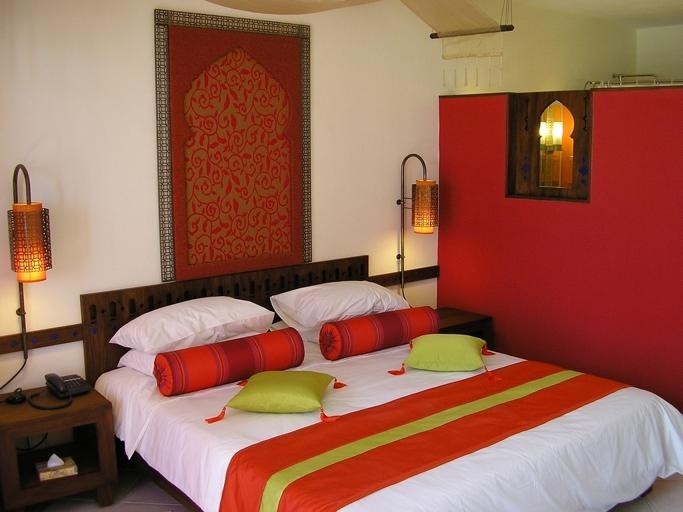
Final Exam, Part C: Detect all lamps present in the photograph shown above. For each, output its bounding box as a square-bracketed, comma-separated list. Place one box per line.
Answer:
[7, 164, 53, 358]
[397, 154, 439, 288]
[539, 122, 564, 152]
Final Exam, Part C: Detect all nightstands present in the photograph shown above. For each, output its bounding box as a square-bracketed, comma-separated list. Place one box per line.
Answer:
[0, 387, 119, 512]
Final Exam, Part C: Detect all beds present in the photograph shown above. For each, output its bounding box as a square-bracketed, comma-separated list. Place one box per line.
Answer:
[79, 256, 683, 512]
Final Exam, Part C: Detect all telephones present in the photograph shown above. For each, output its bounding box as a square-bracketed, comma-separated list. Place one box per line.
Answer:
[44, 373, 92, 399]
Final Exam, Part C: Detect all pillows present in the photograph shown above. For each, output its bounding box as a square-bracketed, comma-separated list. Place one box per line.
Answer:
[386, 332, 494, 376]
[204, 364, 349, 424]
[270, 320, 290, 333]
[269, 281, 411, 345]
[116, 346, 156, 378]
[320, 302, 441, 361]
[108, 292, 275, 354]
[151, 324, 306, 398]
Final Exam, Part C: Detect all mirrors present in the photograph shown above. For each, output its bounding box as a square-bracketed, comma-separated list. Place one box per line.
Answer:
[538, 101, 574, 188]
